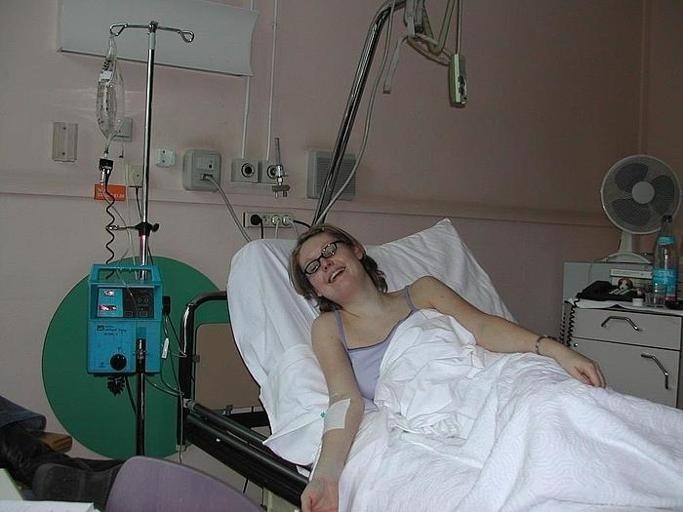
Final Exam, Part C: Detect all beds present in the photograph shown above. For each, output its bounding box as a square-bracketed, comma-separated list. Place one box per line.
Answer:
[175, 218, 683, 512]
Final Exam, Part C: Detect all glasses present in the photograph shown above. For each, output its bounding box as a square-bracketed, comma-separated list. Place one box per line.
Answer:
[302, 240, 343, 275]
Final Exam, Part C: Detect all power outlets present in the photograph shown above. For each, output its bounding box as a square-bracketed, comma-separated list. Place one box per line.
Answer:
[243, 211, 295, 228]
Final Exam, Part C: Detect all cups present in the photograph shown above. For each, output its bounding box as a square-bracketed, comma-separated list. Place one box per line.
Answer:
[642, 281, 668, 308]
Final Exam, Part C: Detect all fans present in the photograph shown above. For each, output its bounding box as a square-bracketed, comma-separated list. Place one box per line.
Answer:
[593, 153, 682, 265]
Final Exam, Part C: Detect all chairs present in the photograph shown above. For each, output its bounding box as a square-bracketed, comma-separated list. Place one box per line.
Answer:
[104, 455, 266, 512]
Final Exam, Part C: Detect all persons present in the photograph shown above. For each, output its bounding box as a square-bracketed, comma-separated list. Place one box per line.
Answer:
[290, 224, 607, 511]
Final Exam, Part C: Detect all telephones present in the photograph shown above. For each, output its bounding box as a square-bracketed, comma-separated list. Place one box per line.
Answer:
[582, 280, 645, 302]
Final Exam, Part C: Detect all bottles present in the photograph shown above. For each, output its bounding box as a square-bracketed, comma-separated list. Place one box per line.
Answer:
[654, 214, 678, 301]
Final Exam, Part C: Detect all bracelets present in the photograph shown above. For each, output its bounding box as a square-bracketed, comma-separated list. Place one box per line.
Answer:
[536, 332, 556, 357]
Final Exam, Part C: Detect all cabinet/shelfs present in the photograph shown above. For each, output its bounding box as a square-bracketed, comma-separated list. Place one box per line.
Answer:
[560, 300, 683, 410]
